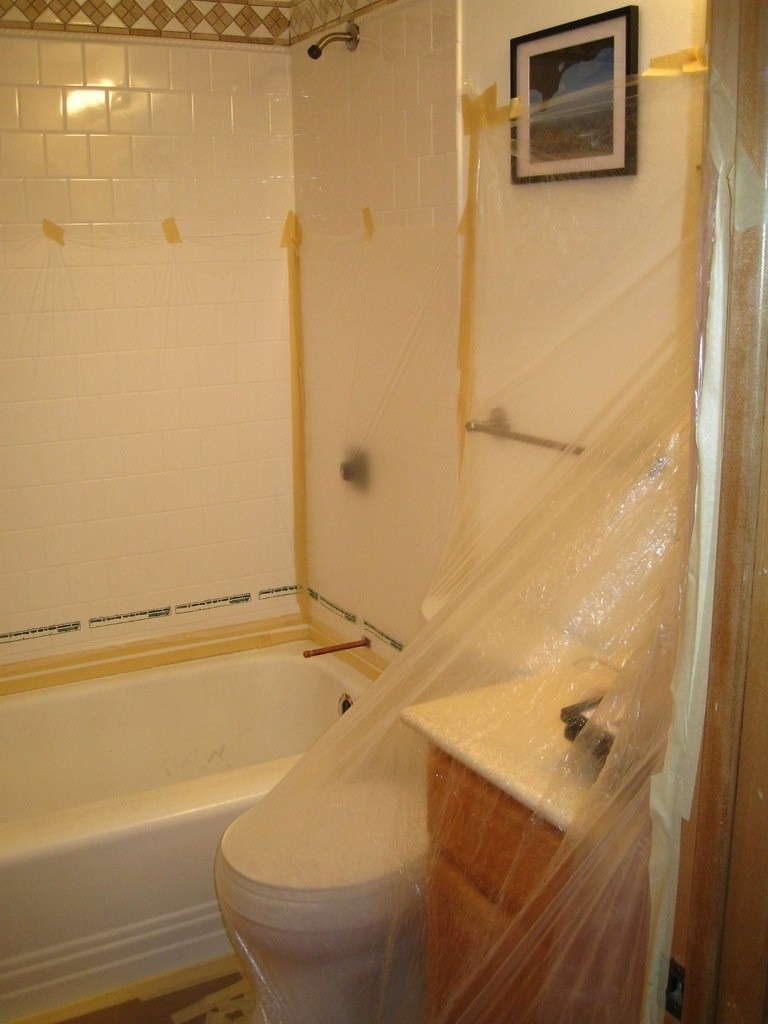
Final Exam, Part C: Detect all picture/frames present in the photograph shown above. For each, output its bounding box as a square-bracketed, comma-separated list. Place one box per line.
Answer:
[509, 5, 640, 183]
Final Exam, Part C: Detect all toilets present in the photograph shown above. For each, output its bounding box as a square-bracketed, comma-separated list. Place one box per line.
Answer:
[213, 778, 430, 1024]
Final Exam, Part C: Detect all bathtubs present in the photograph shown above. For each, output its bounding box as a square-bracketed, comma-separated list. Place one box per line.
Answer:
[0, 639, 392, 1024]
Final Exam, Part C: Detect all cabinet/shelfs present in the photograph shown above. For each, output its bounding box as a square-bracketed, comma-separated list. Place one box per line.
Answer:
[400, 671, 655, 1024]
